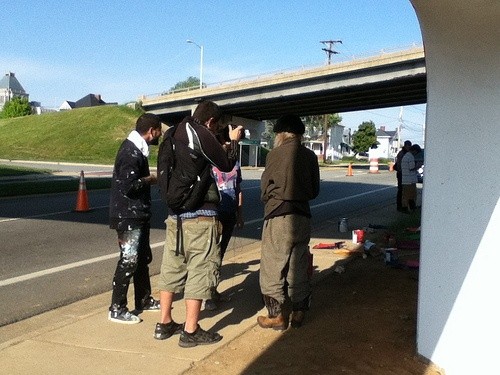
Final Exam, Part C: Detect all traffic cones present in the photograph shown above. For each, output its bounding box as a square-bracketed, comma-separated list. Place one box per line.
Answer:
[388, 161, 394, 172]
[72, 169, 94, 212]
[345, 163, 354, 176]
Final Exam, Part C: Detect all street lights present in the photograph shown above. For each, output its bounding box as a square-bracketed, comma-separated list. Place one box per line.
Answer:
[186, 39, 204, 89]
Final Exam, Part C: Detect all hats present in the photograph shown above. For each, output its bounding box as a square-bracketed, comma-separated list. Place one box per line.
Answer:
[273, 115, 305, 135]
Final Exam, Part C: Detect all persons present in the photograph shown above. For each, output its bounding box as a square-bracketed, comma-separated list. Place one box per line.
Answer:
[152, 99, 243, 348]
[393, 140, 412, 211]
[257, 113, 320, 330]
[109, 113, 161, 324]
[401, 144, 421, 212]
[202, 123, 242, 310]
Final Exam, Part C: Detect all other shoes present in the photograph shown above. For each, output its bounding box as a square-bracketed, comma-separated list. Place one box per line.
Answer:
[258, 313, 286, 330]
[205, 294, 231, 310]
[410, 206, 420, 210]
[154, 319, 184, 340]
[396, 207, 402, 210]
[291, 311, 305, 328]
[136, 298, 160, 311]
[108, 311, 141, 324]
[403, 207, 408, 212]
[179, 324, 220, 347]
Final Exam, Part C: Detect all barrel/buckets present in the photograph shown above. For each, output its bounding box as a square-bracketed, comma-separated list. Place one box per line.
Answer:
[384, 247, 400, 268]
[351, 229, 364, 244]
[337, 217, 349, 233]
[362, 240, 379, 258]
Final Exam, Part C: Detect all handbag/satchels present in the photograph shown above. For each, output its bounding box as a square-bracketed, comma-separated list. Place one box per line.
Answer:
[236, 206, 244, 229]
